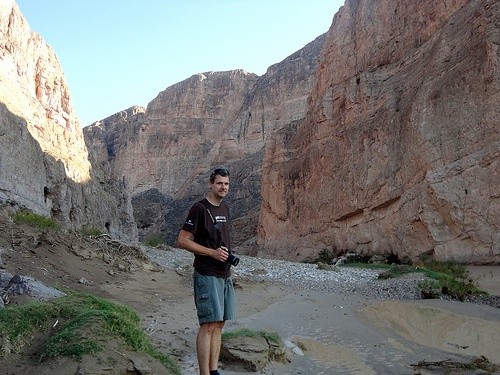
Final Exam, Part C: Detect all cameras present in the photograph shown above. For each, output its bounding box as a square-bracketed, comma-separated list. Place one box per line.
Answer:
[220, 250, 240, 271]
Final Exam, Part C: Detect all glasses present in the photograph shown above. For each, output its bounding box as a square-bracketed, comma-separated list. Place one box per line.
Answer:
[212, 169, 229, 177]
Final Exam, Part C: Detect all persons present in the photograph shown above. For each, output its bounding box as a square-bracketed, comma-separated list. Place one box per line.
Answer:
[178, 169, 235, 375]
